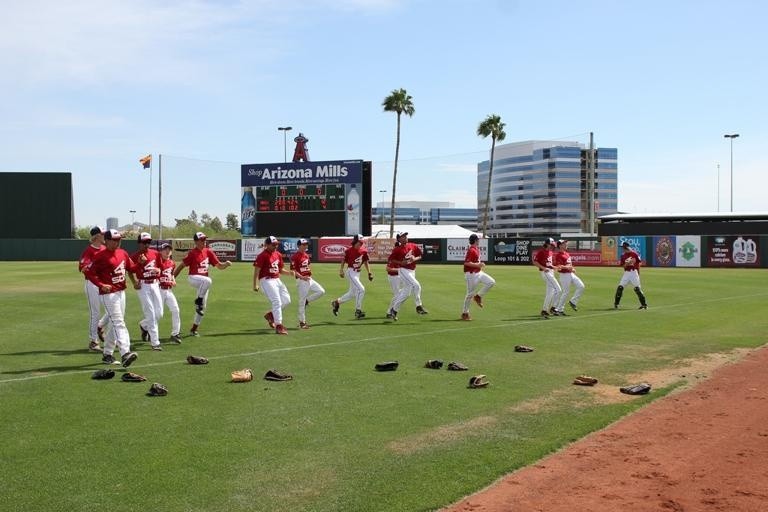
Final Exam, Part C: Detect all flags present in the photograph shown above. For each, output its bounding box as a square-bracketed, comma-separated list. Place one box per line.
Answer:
[140, 154, 151, 169]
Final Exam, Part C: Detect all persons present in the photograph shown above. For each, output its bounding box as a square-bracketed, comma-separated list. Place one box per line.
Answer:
[390, 232, 429, 321]
[556, 240, 585, 315]
[615, 242, 648, 311]
[174, 231, 232, 337]
[332, 234, 373, 318]
[535, 238, 562, 319]
[461, 234, 495, 321]
[290, 239, 325, 330]
[79, 226, 181, 368]
[386, 242, 401, 321]
[253, 236, 295, 335]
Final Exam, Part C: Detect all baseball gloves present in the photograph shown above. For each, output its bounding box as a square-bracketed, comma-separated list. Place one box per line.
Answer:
[122, 372, 146, 382]
[148, 383, 168, 397]
[188, 355, 210, 365]
[374, 360, 398, 371]
[470, 374, 491, 388]
[515, 345, 533, 352]
[231, 369, 254, 382]
[575, 374, 599, 385]
[619, 382, 652, 394]
[448, 362, 468, 371]
[425, 360, 443, 368]
[264, 369, 292, 380]
[92, 369, 114, 380]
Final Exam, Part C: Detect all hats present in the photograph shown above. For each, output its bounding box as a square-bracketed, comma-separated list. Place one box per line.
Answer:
[265, 236, 281, 245]
[469, 234, 481, 243]
[89, 226, 172, 249]
[193, 231, 208, 240]
[297, 238, 309, 248]
[617, 241, 628, 247]
[353, 234, 368, 243]
[395, 241, 402, 247]
[544, 237, 557, 248]
[396, 231, 408, 241]
[556, 239, 568, 248]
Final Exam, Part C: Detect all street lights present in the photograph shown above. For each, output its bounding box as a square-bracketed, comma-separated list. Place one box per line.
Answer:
[129, 210, 136, 225]
[723, 133, 740, 214]
[380, 190, 387, 224]
[278, 127, 292, 163]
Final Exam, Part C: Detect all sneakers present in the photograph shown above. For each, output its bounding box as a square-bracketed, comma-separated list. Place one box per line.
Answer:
[386, 314, 392, 318]
[140, 325, 182, 351]
[415, 305, 428, 314]
[299, 321, 309, 330]
[331, 300, 340, 316]
[389, 308, 398, 321]
[473, 295, 483, 308]
[264, 312, 275, 328]
[354, 309, 365, 318]
[461, 313, 472, 321]
[541, 302, 578, 319]
[195, 297, 203, 316]
[89, 326, 138, 367]
[275, 325, 287, 335]
[189, 327, 200, 338]
